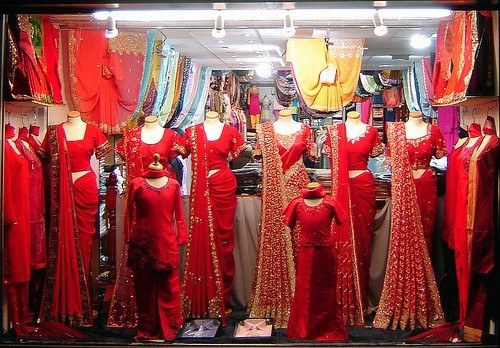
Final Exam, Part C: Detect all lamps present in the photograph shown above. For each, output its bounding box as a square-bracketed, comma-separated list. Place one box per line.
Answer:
[211, 16, 227, 40]
[103, 16, 120, 40]
[371, 14, 388, 38]
[283, 16, 297, 37]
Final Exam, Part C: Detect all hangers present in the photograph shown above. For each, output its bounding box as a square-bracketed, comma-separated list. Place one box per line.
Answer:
[6, 109, 38, 132]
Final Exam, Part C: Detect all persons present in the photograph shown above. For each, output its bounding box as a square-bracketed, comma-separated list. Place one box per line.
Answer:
[248, 85, 261, 128]
[246, 109, 317, 328]
[323, 109, 385, 328]
[125, 153, 190, 342]
[39, 110, 113, 328]
[4, 135, 31, 335]
[175, 110, 248, 319]
[261, 93, 271, 123]
[466, 120, 500, 276]
[28, 131, 51, 239]
[371, 111, 449, 331]
[453, 122, 484, 323]
[14, 136, 49, 272]
[442, 125, 470, 252]
[107, 115, 184, 329]
[281, 181, 351, 342]
